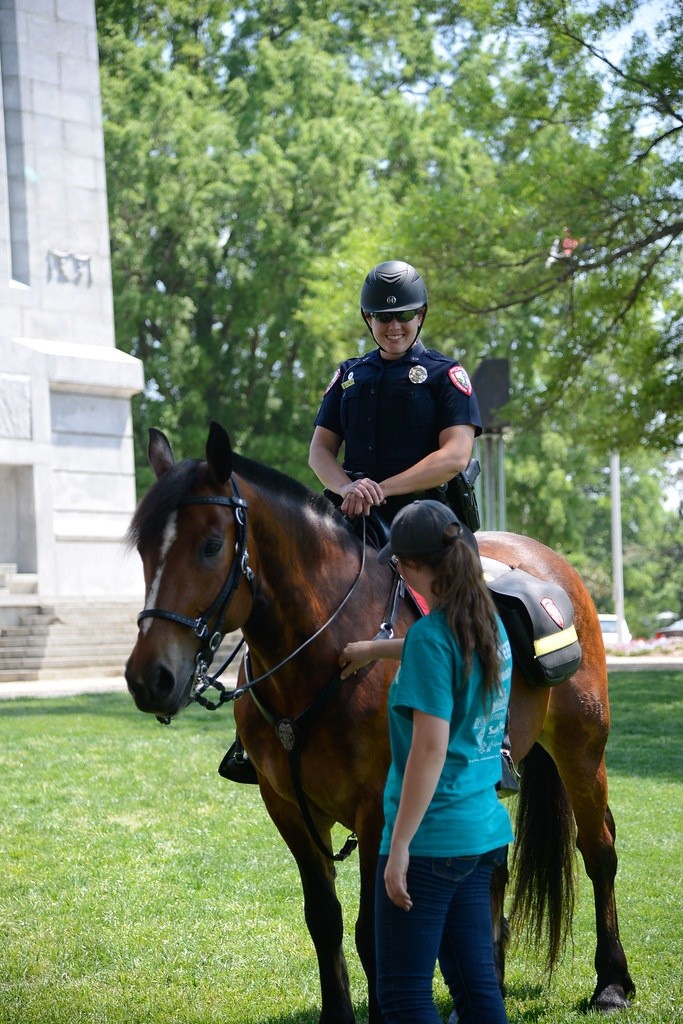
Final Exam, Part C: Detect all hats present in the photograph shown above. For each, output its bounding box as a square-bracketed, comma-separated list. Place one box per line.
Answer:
[376, 500, 462, 563]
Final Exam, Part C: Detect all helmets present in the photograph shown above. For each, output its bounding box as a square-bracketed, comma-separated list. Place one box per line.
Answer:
[360, 261, 428, 315]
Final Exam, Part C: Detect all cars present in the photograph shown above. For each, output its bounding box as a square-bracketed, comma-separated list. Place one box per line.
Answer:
[654, 618, 683, 638]
[597, 612, 631, 654]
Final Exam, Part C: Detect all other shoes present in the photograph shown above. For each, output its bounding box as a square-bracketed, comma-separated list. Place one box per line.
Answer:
[497, 707, 521, 798]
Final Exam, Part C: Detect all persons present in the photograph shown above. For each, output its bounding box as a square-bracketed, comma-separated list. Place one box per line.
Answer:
[339, 500, 514, 1024]
[227, 260, 521, 799]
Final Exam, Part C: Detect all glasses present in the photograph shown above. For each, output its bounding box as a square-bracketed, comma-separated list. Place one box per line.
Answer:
[365, 306, 426, 323]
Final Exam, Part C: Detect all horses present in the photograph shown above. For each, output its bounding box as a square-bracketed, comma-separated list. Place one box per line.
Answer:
[124, 420, 638, 1024]
